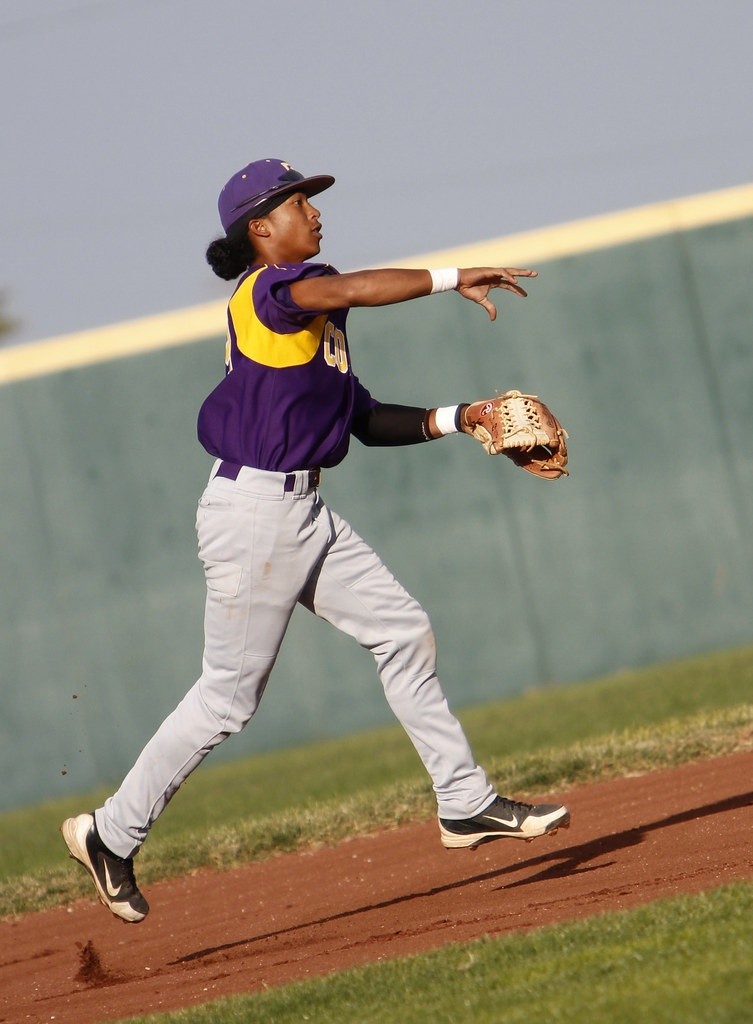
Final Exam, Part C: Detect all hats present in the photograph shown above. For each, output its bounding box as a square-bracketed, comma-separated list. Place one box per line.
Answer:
[218, 158, 336, 232]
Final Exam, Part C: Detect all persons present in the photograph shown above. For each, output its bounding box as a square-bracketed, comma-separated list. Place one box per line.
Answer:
[60, 157, 567, 922]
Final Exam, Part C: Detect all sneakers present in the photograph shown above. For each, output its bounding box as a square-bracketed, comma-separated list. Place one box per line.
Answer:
[437, 795, 570, 848]
[58, 810, 150, 924]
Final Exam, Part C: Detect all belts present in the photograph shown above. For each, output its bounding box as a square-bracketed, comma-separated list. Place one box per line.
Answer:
[215, 460, 321, 492]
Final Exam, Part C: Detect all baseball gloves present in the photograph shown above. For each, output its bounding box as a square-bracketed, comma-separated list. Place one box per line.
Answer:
[462, 389, 568, 480]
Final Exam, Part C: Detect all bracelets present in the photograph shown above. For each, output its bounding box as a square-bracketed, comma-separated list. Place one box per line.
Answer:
[435, 405, 458, 435]
[428, 268, 458, 294]
[455, 403, 470, 432]
